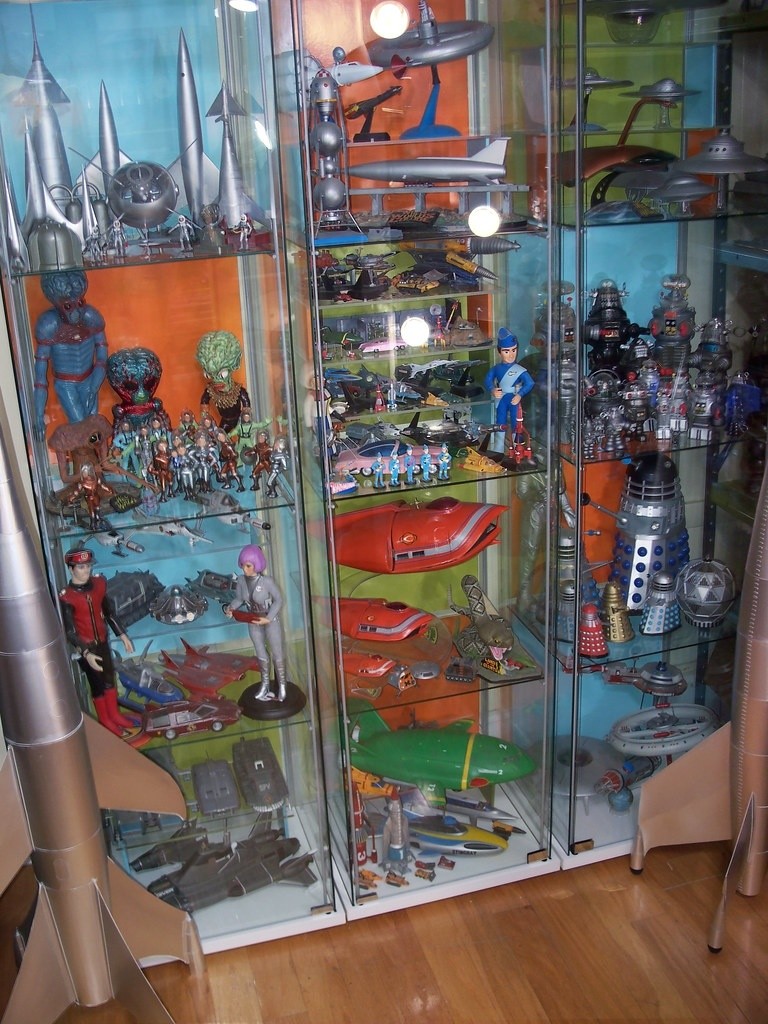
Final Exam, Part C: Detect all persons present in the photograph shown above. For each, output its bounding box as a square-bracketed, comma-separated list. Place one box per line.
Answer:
[59, 548, 135, 735]
[517, 438, 580, 616]
[225, 545, 289, 702]
[167, 215, 196, 252]
[108, 219, 128, 258]
[485, 328, 536, 447]
[85, 223, 106, 266]
[112, 407, 289, 502]
[299, 363, 338, 480]
[232, 214, 253, 251]
[64, 463, 119, 530]
[370, 442, 452, 489]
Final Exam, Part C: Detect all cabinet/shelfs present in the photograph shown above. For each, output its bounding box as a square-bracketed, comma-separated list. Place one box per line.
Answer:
[3, 0, 345, 978]
[268, 2, 569, 926]
[476, 5, 768, 872]
